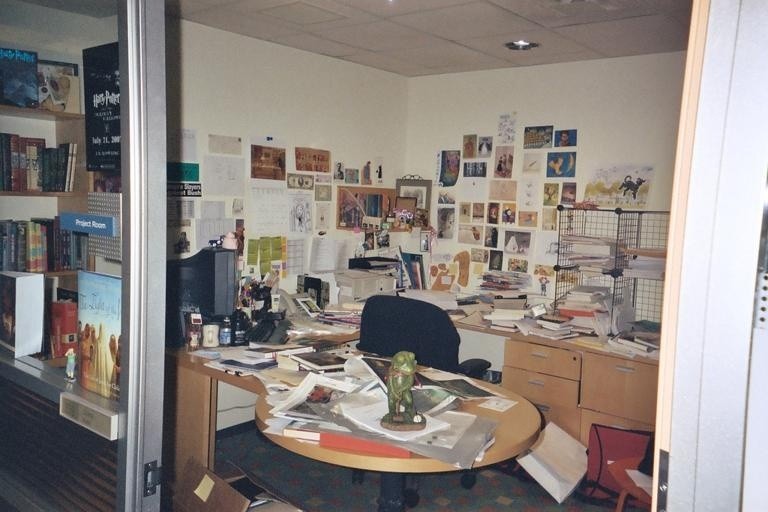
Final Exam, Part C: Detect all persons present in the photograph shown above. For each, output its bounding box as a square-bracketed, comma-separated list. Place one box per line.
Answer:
[363, 161, 371, 185]
[333, 162, 344, 180]
[175, 231, 189, 253]
[412, 126, 576, 296]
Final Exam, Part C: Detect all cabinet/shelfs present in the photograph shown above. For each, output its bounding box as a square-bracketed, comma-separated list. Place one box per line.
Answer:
[0, 105, 96, 292]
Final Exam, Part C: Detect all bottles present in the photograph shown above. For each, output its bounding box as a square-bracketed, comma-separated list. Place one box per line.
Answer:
[219, 316, 244, 347]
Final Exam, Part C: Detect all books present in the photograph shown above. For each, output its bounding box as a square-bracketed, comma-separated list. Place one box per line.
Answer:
[229, 477, 272, 509]
[73, 267, 123, 398]
[607, 319, 660, 357]
[0, 133, 78, 192]
[0, 217, 89, 274]
[217, 337, 329, 377]
[1, 47, 83, 115]
[259, 347, 505, 469]
[318, 302, 365, 331]
[444, 286, 612, 341]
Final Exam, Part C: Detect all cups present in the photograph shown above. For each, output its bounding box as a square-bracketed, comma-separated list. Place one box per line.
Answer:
[203, 324, 219, 348]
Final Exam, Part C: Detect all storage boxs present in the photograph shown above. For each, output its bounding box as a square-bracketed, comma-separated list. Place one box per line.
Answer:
[174, 455, 308, 512]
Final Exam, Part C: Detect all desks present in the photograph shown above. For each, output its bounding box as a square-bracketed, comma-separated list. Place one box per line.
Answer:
[161, 321, 659, 512]
[255, 366, 542, 512]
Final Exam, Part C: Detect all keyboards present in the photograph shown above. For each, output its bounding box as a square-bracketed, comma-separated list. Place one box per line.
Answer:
[244, 319, 276, 342]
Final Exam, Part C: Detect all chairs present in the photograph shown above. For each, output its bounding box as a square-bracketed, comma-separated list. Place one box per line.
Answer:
[351, 296, 491, 508]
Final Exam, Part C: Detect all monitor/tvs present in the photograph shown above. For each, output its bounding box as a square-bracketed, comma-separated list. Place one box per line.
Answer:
[166, 246, 236, 323]
